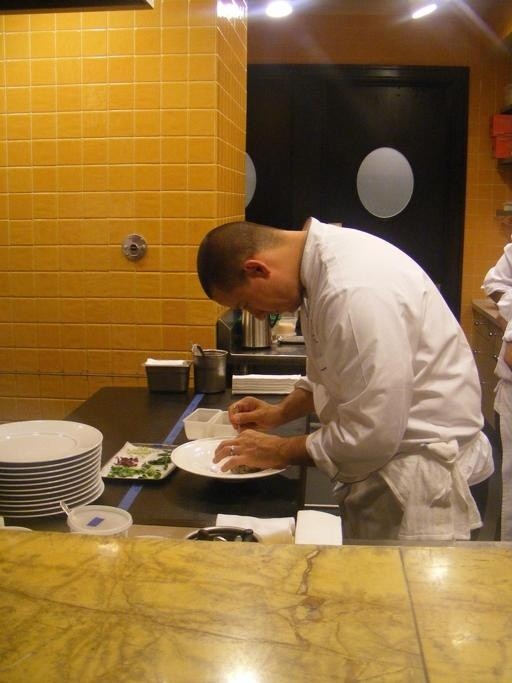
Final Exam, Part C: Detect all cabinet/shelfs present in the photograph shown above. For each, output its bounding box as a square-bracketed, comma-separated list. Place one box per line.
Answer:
[247, 64, 470, 325]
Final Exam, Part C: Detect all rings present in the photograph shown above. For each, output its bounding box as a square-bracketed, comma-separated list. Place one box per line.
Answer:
[230, 445, 235, 456]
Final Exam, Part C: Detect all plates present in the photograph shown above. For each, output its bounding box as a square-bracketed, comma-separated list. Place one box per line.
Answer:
[0, 420, 105, 523]
[171, 435, 294, 484]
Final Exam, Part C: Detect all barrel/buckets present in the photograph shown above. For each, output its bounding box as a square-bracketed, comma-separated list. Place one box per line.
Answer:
[191, 349, 228, 395]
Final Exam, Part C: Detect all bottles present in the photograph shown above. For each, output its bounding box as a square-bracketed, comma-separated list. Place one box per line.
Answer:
[241, 308, 280, 348]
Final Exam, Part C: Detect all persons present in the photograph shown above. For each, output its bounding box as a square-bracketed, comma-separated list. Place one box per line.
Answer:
[197, 216, 495, 547]
[479, 231, 512, 544]
